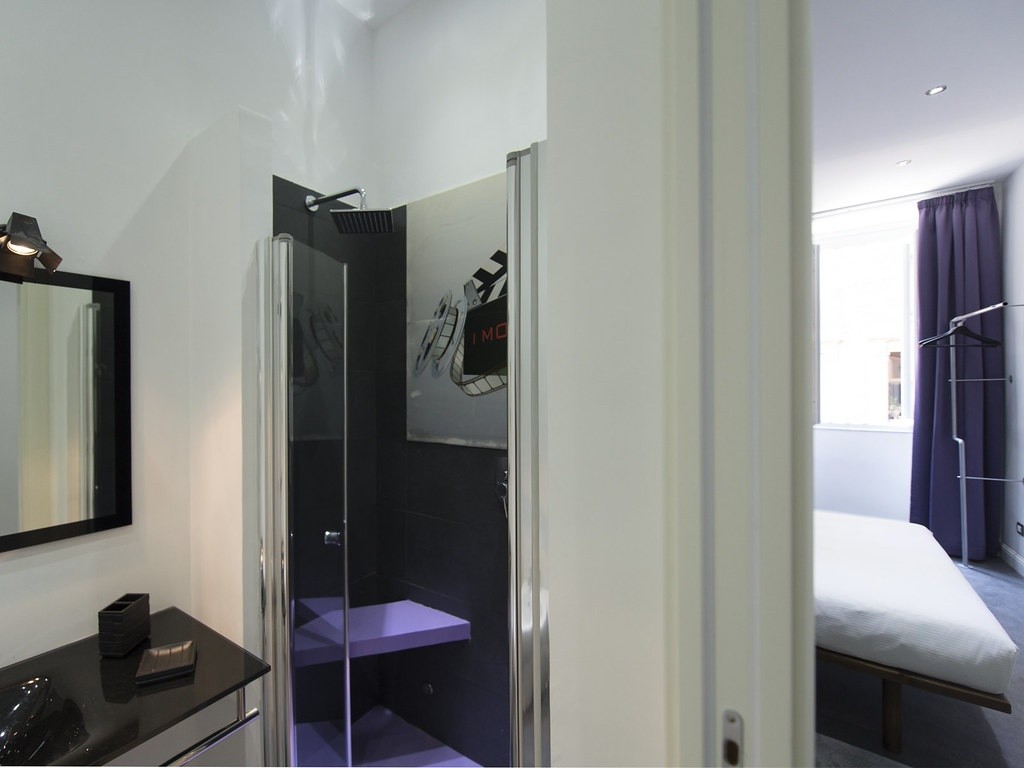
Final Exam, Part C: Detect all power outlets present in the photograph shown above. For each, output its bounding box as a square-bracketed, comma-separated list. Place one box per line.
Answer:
[1016, 523, 1024, 535]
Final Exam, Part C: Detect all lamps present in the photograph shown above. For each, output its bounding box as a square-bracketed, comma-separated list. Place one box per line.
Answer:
[0, 212, 63, 277]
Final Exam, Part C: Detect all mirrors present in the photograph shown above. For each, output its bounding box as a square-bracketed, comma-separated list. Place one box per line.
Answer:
[0, 268, 132, 552]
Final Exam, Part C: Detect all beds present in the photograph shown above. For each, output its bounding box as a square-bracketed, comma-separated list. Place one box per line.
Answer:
[811, 510, 1019, 756]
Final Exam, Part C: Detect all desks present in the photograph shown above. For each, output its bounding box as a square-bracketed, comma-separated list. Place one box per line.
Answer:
[0, 605, 271, 767]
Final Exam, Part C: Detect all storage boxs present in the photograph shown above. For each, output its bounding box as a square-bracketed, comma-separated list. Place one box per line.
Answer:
[99, 593, 150, 658]
